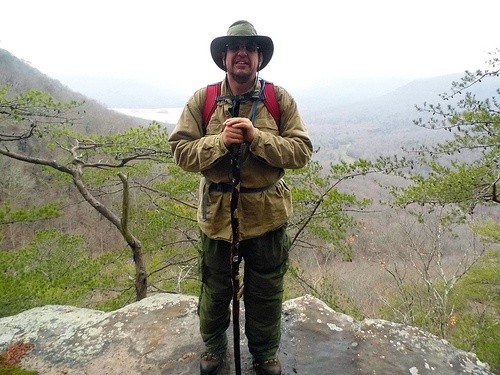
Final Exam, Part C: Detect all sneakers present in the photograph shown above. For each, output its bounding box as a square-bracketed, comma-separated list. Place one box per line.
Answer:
[199, 351, 221, 375]
[257, 355, 282, 375]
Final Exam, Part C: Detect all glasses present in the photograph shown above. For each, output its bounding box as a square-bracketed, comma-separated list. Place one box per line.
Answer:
[226, 41, 261, 52]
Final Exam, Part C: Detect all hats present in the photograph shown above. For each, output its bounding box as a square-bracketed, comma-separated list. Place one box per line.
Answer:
[210, 20, 274, 72]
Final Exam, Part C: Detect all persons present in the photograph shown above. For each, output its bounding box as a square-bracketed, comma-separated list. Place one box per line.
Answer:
[167, 19, 312, 375]
